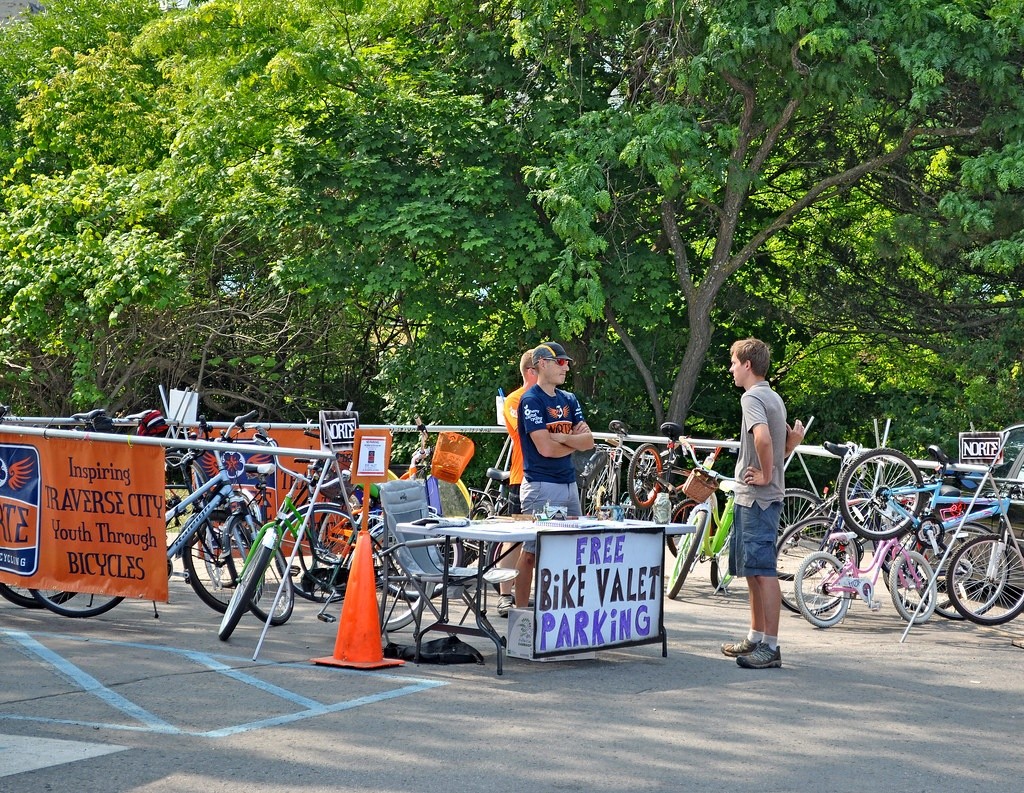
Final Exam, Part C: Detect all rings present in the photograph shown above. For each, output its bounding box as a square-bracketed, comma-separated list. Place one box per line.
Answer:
[749, 476, 753, 480]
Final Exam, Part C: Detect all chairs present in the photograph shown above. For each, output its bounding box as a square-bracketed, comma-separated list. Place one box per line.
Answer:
[379, 479, 520, 645]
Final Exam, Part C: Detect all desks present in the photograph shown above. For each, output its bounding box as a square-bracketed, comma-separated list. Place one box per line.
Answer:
[396, 516, 696, 675]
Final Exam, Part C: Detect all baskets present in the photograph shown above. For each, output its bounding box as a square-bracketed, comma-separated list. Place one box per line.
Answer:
[570, 447, 608, 490]
[430, 432, 475, 484]
[320, 460, 358, 506]
[681, 467, 720, 504]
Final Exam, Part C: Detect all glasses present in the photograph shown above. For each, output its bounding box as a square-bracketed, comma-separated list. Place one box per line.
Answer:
[544, 358, 570, 366]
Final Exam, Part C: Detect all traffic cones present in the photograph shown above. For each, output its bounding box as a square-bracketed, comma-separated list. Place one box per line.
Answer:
[310, 530, 406, 671]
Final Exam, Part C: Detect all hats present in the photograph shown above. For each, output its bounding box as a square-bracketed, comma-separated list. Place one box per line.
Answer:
[532, 342, 573, 366]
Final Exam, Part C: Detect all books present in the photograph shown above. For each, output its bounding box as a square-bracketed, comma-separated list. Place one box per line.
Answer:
[535, 518, 606, 528]
[409, 515, 470, 530]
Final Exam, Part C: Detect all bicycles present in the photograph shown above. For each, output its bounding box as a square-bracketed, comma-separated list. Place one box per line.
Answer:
[0, 396, 1024, 632]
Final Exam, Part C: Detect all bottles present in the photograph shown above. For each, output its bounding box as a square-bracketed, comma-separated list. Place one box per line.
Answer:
[702, 452, 715, 472]
[653, 492, 671, 523]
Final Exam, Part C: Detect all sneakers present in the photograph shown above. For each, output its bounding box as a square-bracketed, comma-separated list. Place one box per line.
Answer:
[497, 594, 514, 617]
[720, 636, 762, 657]
[736, 641, 782, 669]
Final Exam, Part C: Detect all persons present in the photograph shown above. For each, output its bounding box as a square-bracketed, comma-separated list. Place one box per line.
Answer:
[497, 342, 594, 617]
[721, 338, 804, 669]
